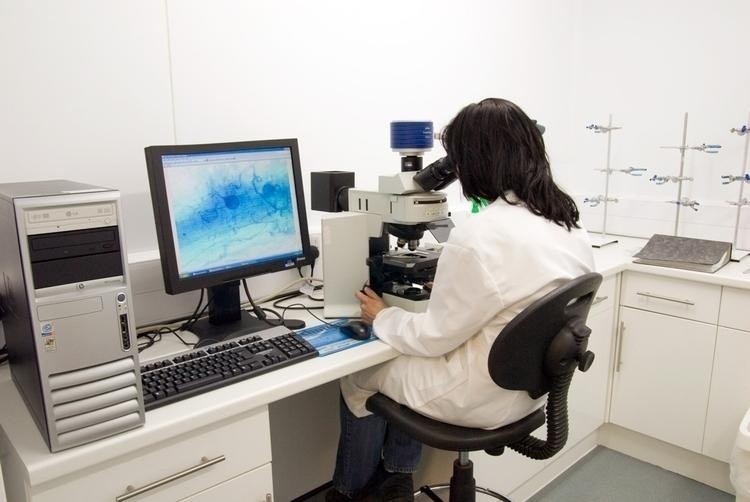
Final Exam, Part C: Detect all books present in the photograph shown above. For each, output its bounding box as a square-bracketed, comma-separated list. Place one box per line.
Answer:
[632, 234, 732, 273]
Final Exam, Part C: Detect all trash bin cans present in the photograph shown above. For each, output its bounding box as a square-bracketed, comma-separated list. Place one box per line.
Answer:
[730, 409, 750, 502]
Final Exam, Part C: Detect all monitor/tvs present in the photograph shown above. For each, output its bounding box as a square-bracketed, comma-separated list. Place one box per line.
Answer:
[145, 138, 312, 344]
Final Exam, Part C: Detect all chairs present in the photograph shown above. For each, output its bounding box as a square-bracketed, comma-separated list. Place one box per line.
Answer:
[361, 272, 610, 502]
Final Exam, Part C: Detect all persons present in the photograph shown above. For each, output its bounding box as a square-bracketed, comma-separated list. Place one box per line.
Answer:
[326, 98, 594, 502]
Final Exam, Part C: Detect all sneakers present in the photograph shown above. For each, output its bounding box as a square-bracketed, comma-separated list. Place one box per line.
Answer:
[325, 471, 414, 502]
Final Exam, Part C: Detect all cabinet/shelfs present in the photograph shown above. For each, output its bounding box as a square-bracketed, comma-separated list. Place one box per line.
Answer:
[597, 258, 750, 491]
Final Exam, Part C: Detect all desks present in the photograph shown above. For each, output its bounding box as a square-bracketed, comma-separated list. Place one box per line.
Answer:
[1, 265, 623, 501]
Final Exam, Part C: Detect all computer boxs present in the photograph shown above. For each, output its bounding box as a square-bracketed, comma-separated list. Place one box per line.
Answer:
[2, 180, 146, 453]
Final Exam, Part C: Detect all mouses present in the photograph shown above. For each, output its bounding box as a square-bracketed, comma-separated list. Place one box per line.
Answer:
[338, 322, 372, 339]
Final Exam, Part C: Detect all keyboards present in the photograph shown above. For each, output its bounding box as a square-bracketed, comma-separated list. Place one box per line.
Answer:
[141, 326, 319, 410]
